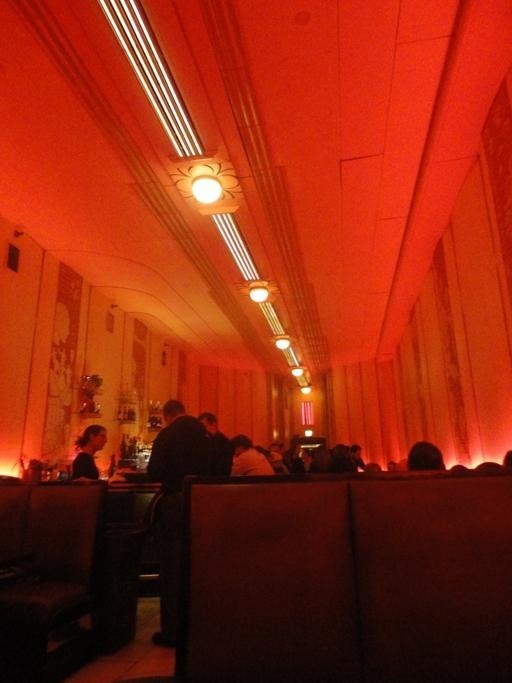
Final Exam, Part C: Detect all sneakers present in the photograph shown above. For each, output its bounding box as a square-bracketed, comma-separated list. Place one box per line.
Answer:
[152, 631, 176, 647]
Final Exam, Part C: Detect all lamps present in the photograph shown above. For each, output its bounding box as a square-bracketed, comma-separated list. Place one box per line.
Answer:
[160, 152, 245, 220]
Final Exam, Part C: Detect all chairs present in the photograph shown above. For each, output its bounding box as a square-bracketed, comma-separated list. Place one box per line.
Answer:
[187, 476, 512, 679]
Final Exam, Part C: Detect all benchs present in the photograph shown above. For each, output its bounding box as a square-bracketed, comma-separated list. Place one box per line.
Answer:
[2, 480, 107, 681]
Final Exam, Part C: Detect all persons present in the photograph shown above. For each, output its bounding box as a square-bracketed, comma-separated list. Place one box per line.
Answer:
[70, 424, 108, 480]
[146, 399, 460, 648]
